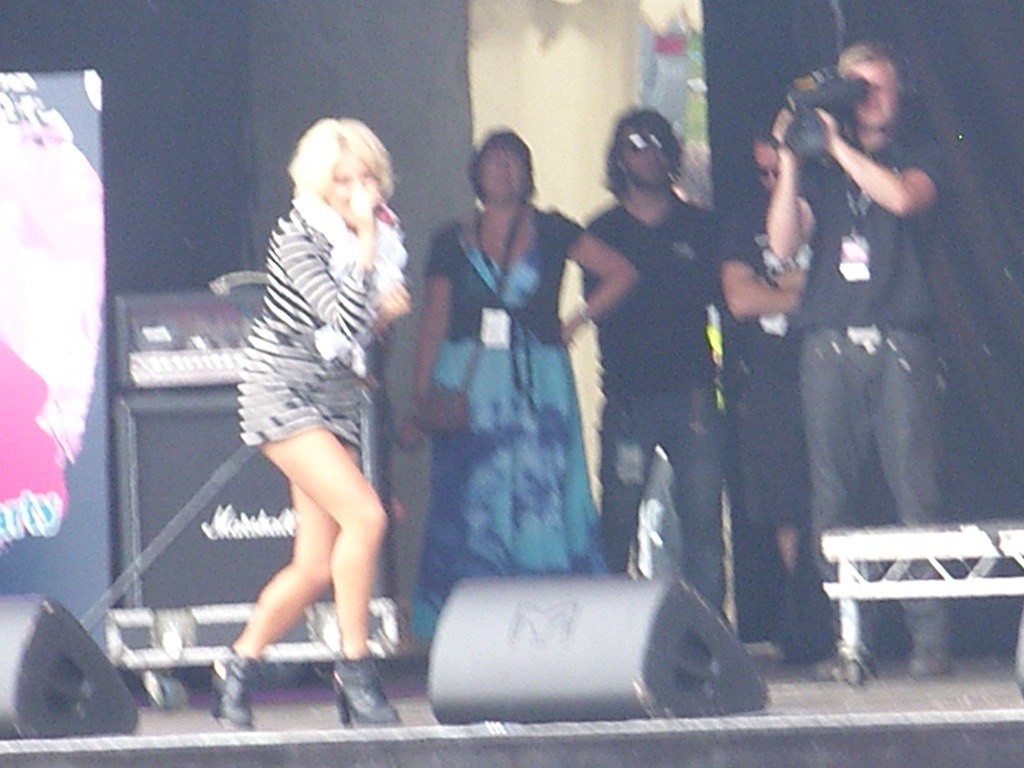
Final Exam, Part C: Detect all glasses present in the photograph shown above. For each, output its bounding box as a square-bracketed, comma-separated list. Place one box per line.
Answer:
[620, 132, 664, 150]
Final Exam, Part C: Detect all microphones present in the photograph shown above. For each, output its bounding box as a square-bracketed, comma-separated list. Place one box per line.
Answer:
[371, 198, 403, 231]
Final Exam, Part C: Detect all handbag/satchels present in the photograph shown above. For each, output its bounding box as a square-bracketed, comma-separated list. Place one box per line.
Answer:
[414, 386, 471, 436]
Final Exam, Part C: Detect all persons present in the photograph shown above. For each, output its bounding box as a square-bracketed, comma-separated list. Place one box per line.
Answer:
[765, 39, 961, 677]
[720, 119, 838, 672]
[583, 108, 728, 623]
[410, 128, 643, 647]
[208, 116, 411, 732]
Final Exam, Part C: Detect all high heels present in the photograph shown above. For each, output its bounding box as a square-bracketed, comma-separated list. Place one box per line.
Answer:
[208, 647, 260, 731]
[331, 653, 404, 728]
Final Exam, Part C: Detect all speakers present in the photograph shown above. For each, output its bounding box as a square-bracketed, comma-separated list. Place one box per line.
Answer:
[426, 577, 770, 724]
[0, 594, 142, 743]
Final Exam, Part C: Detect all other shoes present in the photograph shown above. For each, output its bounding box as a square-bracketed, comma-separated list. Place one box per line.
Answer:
[903, 650, 954, 679]
[815, 647, 876, 688]
